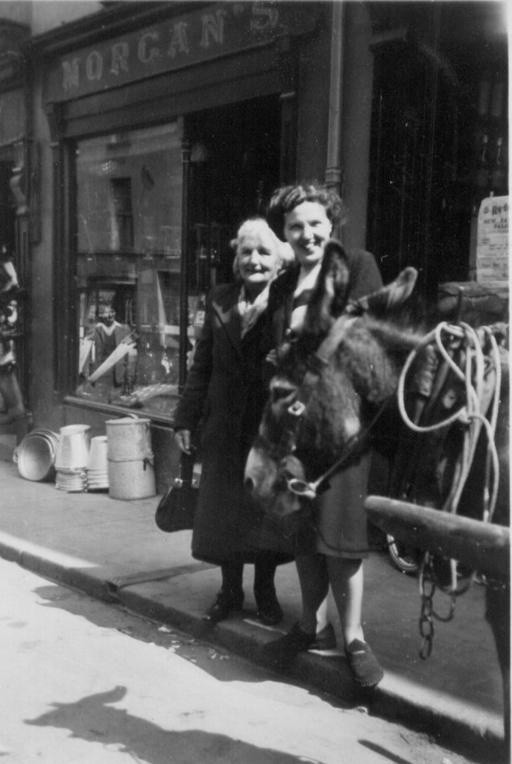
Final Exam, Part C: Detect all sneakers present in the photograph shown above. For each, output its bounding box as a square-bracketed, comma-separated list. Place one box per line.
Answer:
[344, 639, 384, 688]
[264, 620, 336, 652]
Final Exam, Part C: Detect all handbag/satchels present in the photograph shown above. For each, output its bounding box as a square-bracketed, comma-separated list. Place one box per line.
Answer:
[155, 478, 199, 532]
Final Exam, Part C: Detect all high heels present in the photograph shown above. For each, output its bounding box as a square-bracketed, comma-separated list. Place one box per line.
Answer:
[203, 591, 244, 624]
[254, 586, 283, 626]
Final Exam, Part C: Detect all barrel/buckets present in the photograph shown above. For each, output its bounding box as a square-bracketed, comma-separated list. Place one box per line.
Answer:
[15, 416, 155, 500]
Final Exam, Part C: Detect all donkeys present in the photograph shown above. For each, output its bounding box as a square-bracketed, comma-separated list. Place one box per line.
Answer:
[241, 238, 510, 762]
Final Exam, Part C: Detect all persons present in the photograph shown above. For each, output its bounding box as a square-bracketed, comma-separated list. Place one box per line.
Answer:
[258, 176, 386, 689]
[170, 217, 296, 625]
[80, 299, 207, 405]
